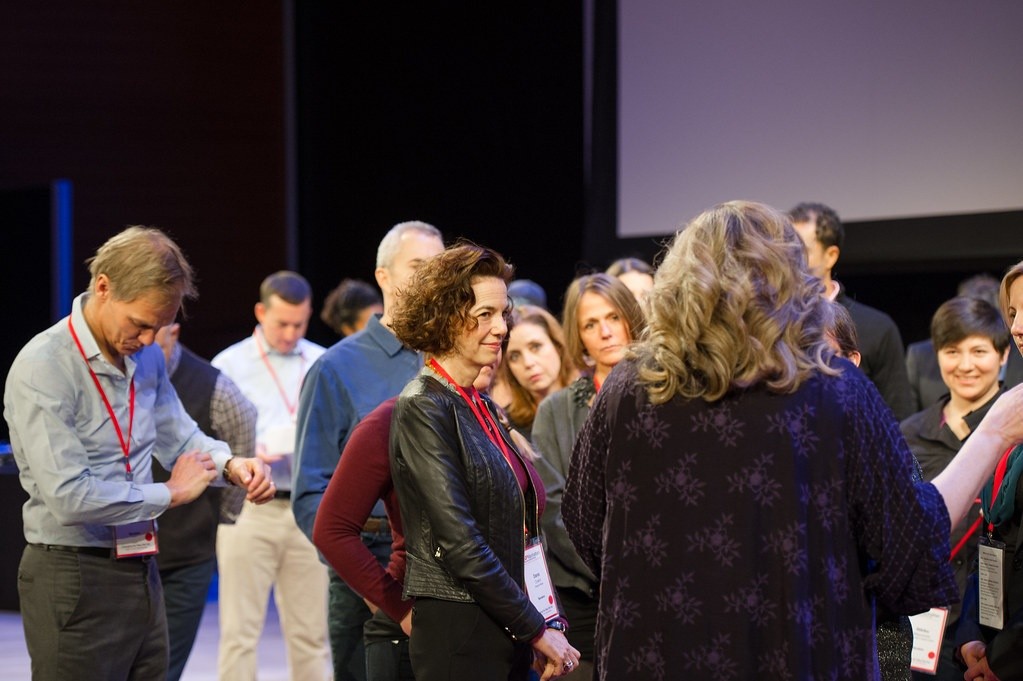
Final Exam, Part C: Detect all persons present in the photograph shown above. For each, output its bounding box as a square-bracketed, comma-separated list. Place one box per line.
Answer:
[789, 204, 914, 424]
[492, 259, 655, 681]
[313, 312, 546, 681]
[292, 223, 444, 681]
[213, 273, 328, 681]
[387, 246, 580, 681]
[152, 322, 258, 681]
[4, 226, 277, 681]
[561, 201, 1023, 681]
[323, 282, 384, 336]
[821, 264, 1023, 681]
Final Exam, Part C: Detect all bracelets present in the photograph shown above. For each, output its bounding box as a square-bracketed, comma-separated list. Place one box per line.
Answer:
[549, 621, 565, 632]
[224, 459, 236, 488]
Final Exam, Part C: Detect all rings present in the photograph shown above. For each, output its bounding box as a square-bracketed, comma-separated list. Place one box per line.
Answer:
[564, 661, 573, 671]
[270, 482, 276, 487]
